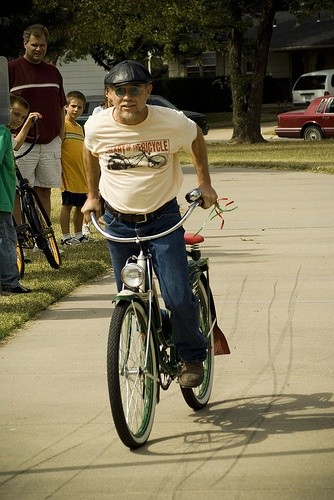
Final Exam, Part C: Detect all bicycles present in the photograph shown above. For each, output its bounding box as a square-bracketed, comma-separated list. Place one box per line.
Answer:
[88, 190, 231, 449]
[11, 115, 61, 278]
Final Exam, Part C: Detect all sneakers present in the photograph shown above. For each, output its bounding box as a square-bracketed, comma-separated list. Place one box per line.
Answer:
[73, 236, 97, 242]
[60, 237, 83, 246]
[2, 284, 32, 292]
[82, 224, 90, 235]
[179, 360, 204, 388]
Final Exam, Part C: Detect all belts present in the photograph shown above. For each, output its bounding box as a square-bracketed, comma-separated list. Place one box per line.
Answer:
[104, 196, 178, 224]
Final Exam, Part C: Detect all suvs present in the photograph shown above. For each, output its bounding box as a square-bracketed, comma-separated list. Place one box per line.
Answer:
[292, 68, 334, 107]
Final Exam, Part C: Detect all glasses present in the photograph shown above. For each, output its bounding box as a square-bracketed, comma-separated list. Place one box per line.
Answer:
[115, 87, 145, 97]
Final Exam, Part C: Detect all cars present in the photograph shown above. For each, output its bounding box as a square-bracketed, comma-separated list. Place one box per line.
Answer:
[275, 95, 333, 141]
[74, 94, 209, 137]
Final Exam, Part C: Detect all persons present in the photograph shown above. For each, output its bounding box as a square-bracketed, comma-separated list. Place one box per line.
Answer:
[8, 23, 68, 264]
[324, 92, 330, 96]
[59, 90, 114, 246]
[80, 59, 218, 390]
[0, 94, 43, 294]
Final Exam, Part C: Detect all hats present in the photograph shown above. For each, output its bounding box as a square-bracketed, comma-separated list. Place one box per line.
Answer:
[104, 60, 152, 87]
